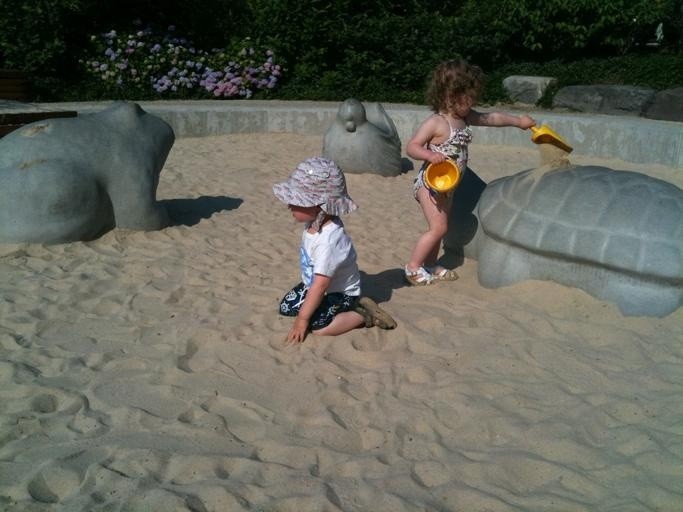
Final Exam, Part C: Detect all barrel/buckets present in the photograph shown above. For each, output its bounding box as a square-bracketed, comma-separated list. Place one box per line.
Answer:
[422, 159, 460, 195]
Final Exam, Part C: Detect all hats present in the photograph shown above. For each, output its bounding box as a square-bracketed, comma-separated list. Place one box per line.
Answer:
[272, 157, 359, 216]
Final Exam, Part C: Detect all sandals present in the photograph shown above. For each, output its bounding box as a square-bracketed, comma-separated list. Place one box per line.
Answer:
[404, 264, 436, 286]
[425, 265, 458, 281]
[354, 296, 396, 330]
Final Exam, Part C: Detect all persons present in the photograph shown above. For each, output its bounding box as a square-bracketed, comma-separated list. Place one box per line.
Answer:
[400, 58, 536, 288]
[272, 154, 396, 347]
